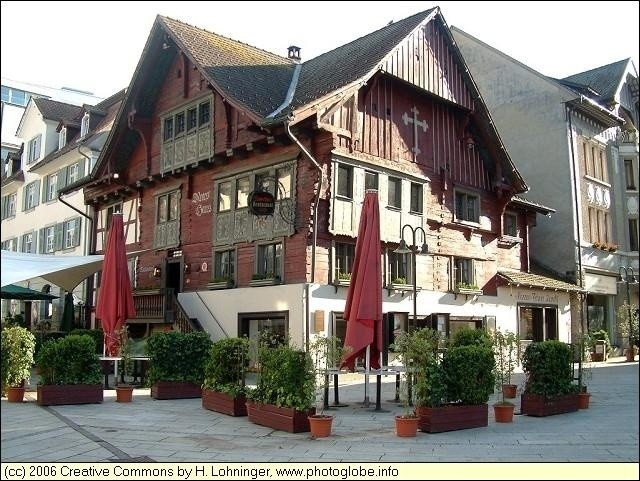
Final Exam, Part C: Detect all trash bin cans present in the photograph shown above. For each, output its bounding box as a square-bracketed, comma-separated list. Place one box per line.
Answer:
[595, 340, 606, 361]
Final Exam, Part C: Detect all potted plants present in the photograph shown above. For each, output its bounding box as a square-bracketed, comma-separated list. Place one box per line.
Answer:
[387, 300, 639, 437]
[0, 323, 353, 438]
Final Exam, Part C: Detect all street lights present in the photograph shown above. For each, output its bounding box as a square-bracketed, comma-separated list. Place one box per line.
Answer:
[614, 261, 638, 361]
[394, 222, 437, 395]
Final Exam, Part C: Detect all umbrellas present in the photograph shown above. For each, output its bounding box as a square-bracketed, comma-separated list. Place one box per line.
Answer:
[95, 211, 138, 386]
[338, 188, 383, 409]
[0, 284, 61, 300]
[1, 244, 178, 293]
